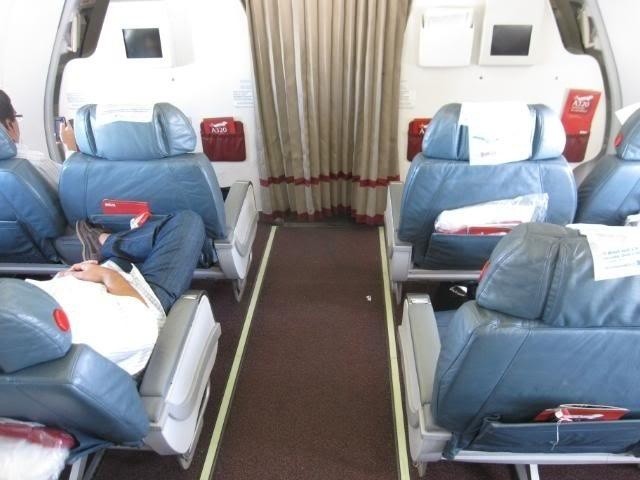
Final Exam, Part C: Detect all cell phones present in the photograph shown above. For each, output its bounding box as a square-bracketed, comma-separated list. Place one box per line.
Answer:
[54, 116, 66, 144]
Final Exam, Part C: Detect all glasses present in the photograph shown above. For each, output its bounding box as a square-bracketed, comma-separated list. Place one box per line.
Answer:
[13, 114, 22, 122]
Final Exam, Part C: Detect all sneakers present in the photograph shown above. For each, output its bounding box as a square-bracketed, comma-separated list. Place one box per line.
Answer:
[75, 219, 111, 263]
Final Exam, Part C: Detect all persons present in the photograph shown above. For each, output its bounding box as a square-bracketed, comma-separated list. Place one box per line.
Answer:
[25, 209, 205, 380]
[0, 90, 77, 191]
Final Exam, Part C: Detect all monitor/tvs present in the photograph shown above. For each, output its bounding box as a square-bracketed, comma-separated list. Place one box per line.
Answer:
[122, 27, 163, 59]
[490, 24, 533, 56]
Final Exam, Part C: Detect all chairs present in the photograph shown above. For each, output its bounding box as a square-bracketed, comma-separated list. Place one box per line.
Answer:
[0, 98, 259, 469]
[384, 96, 639, 464]
